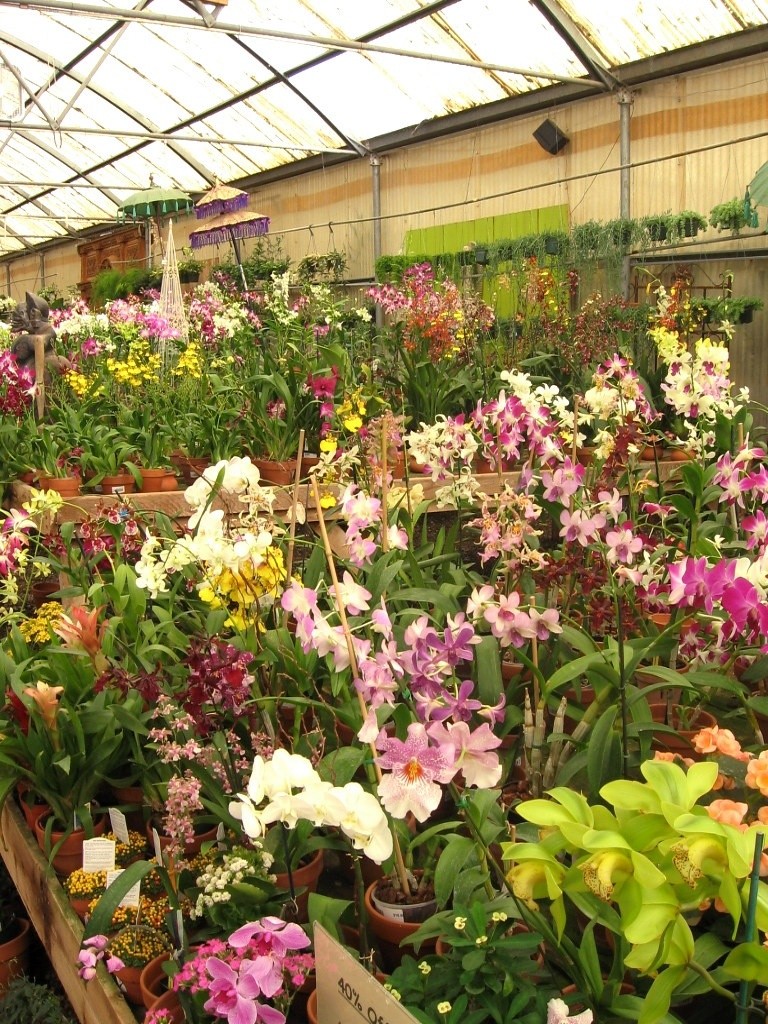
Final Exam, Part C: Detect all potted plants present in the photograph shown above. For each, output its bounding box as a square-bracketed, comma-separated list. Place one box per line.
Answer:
[372, 210, 669, 274]
[669, 210, 706, 241]
[710, 198, 751, 233]
[146, 259, 204, 288]
[212, 230, 348, 281]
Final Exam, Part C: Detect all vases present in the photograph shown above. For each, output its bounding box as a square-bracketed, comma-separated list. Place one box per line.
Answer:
[571, 445, 602, 467]
[292, 920, 357, 981]
[407, 447, 430, 474]
[146, 812, 220, 849]
[168, 453, 183, 474]
[253, 458, 296, 486]
[180, 454, 208, 482]
[641, 438, 663, 461]
[671, 442, 696, 460]
[37, 476, 50, 492]
[364, 868, 443, 935]
[49, 477, 80, 497]
[650, 611, 700, 635]
[106, 934, 141, 1007]
[646, 697, 719, 758]
[140, 948, 180, 1010]
[271, 840, 325, 913]
[144, 986, 189, 1024]
[302, 456, 321, 479]
[67, 890, 100, 913]
[100, 474, 136, 494]
[471, 445, 518, 473]
[114, 783, 139, 804]
[502, 654, 533, 683]
[33, 807, 107, 877]
[17, 786, 47, 830]
[139, 468, 165, 491]
[393, 451, 405, 478]
[0, 918, 32, 989]
[636, 650, 690, 703]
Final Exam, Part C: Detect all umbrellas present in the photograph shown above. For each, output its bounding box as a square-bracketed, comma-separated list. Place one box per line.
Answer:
[116, 175, 193, 260]
[188, 177, 270, 292]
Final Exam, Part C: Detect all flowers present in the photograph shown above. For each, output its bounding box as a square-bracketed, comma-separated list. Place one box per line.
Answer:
[0, 284, 768, 1024]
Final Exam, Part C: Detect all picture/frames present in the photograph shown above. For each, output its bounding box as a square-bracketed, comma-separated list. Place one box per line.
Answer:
[164, 468, 178, 493]
